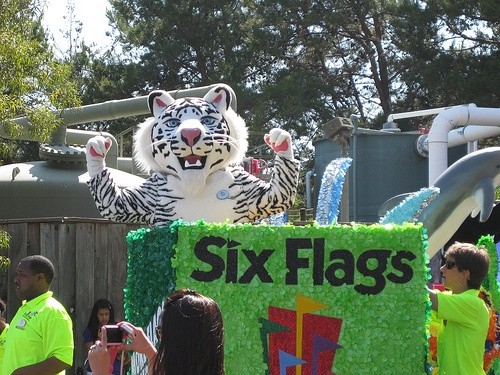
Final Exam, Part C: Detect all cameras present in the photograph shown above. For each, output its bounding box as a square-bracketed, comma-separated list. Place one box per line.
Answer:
[100, 325, 125, 345]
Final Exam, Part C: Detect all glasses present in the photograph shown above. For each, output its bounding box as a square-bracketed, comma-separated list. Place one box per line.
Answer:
[441, 257, 457, 270]
[155, 326, 164, 340]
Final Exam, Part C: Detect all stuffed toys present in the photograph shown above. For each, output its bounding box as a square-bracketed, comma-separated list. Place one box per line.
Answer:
[83, 84, 302, 228]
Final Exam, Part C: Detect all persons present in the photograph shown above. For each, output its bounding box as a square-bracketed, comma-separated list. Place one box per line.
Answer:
[78, 298, 130, 375]
[425, 242, 491, 375]
[88, 289, 226, 375]
[0, 255, 75, 375]
